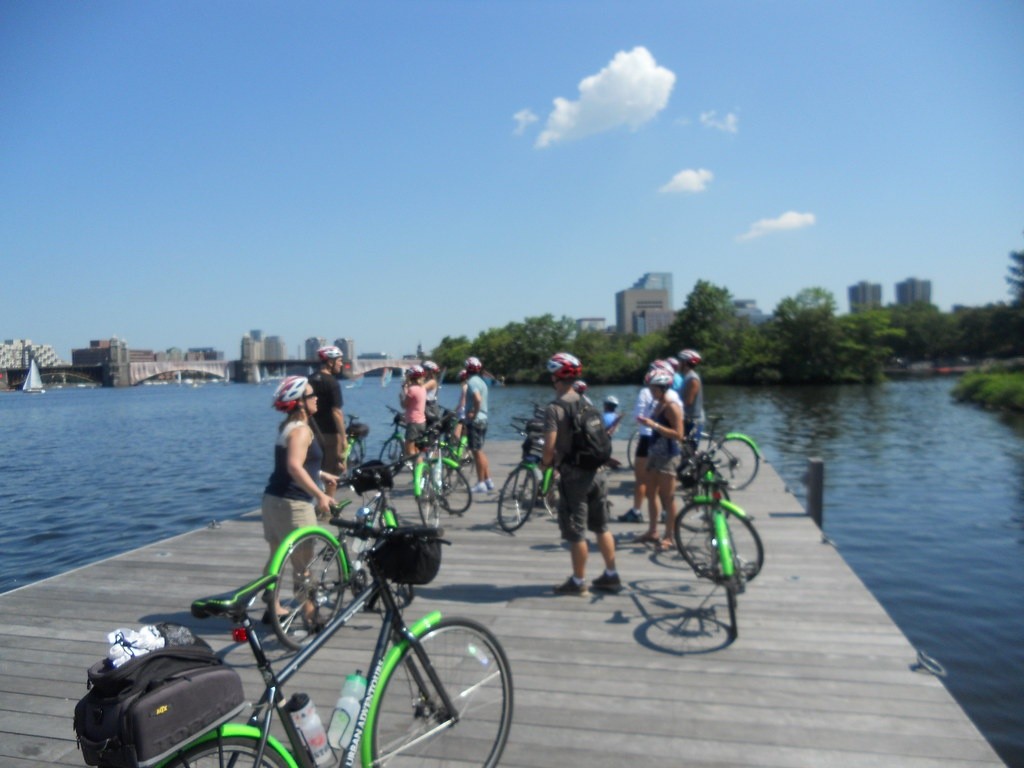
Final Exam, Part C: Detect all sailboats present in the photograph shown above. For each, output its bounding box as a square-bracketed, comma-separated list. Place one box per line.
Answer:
[22, 358, 46, 393]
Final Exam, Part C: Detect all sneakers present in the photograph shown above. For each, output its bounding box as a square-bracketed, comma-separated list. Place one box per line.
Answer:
[470, 482, 488, 492]
[591, 570, 620, 591]
[618, 509, 643, 523]
[554, 577, 584, 595]
[484, 479, 494, 490]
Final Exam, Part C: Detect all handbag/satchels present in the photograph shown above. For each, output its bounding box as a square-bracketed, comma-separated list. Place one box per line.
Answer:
[424, 400, 439, 421]
[656, 436, 683, 459]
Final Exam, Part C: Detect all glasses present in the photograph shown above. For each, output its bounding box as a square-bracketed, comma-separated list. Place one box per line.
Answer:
[305, 390, 316, 399]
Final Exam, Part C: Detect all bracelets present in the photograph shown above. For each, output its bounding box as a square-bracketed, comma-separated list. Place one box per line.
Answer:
[541, 460, 552, 467]
[653, 422, 658, 430]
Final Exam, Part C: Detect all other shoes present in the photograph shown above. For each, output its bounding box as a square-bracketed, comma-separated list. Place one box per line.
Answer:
[656, 536, 678, 551]
[633, 533, 660, 543]
[261, 611, 298, 624]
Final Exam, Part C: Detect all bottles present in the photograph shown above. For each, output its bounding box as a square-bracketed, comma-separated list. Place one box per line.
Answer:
[326, 669, 368, 750]
[283, 692, 337, 768]
[433, 460, 442, 486]
[352, 512, 374, 553]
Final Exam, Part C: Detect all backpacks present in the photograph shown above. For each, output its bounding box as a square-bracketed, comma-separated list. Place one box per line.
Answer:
[549, 393, 612, 463]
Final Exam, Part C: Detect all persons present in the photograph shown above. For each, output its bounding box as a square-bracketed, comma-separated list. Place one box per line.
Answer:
[621, 350, 703, 523]
[463, 356, 495, 495]
[308, 346, 349, 520]
[537, 352, 623, 595]
[400, 360, 440, 475]
[634, 369, 685, 551]
[450, 370, 467, 449]
[259, 375, 339, 632]
[572, 380, 592, 406]
[600, 396, 620, 434]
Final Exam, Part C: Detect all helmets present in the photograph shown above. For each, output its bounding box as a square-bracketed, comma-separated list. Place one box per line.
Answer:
[643, 369, 674, 387]
[405, 365, 425, 379]
[464, 357, 482, 372]
[650, 360, 675, 375]
[316, 345, 344, 362]
[678, 348, 701, 367]
[458, 370, 467, 381]
[666, 356, 679, 367]
[573, 380, 587, 393]
[547, 352, 582, 382]
[271, 375, 308, 413]
[603, 396, 619, 406]
[422, 360, 439, 373]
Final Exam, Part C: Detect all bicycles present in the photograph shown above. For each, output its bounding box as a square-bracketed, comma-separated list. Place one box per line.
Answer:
[669, 449, 767, 641]
[495, 398, 762, 537]
[260, 451, 423, 653]
[68, 513, 514, 768]
[333, 402, 483, 533]
